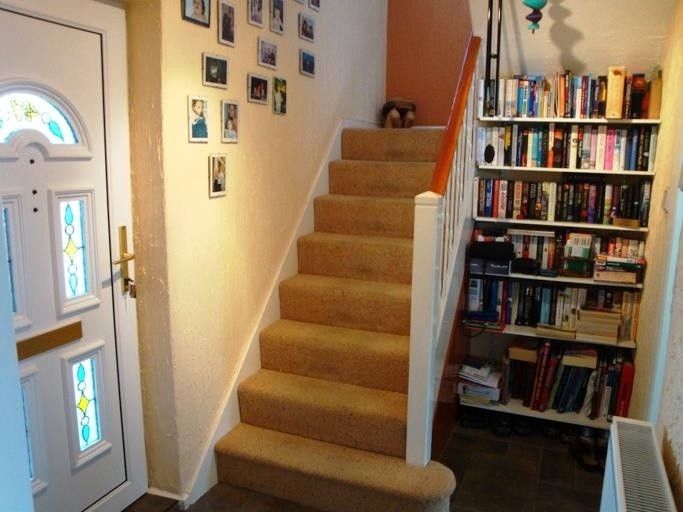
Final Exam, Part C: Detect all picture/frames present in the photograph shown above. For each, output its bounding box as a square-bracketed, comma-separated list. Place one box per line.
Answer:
[175, 1, 323, 200]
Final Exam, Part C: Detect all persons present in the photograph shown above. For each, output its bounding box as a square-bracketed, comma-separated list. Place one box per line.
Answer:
[190, 99, 204, 124]
[215, 160, 224, 191]
[227, 103, 237, 130]
[192, 0, 206, 22]
[209, 1, 318, 114]
[224, 119, 236, 138]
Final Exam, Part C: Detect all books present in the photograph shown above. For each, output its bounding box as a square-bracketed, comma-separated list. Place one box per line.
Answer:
[472, 176, 652, 229]
[477, 122, 658, 174]
[461, 340, 635, 423]
[464, 275, 641, 345]
[507, 228, 645, 283]
[478, 66, 663, 120]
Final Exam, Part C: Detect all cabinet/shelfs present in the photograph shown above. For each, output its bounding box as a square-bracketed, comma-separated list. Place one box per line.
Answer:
[456, 105, 664, 454]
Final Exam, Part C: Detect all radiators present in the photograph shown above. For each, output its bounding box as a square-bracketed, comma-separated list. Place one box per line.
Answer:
[596, 413, 679, 512]
